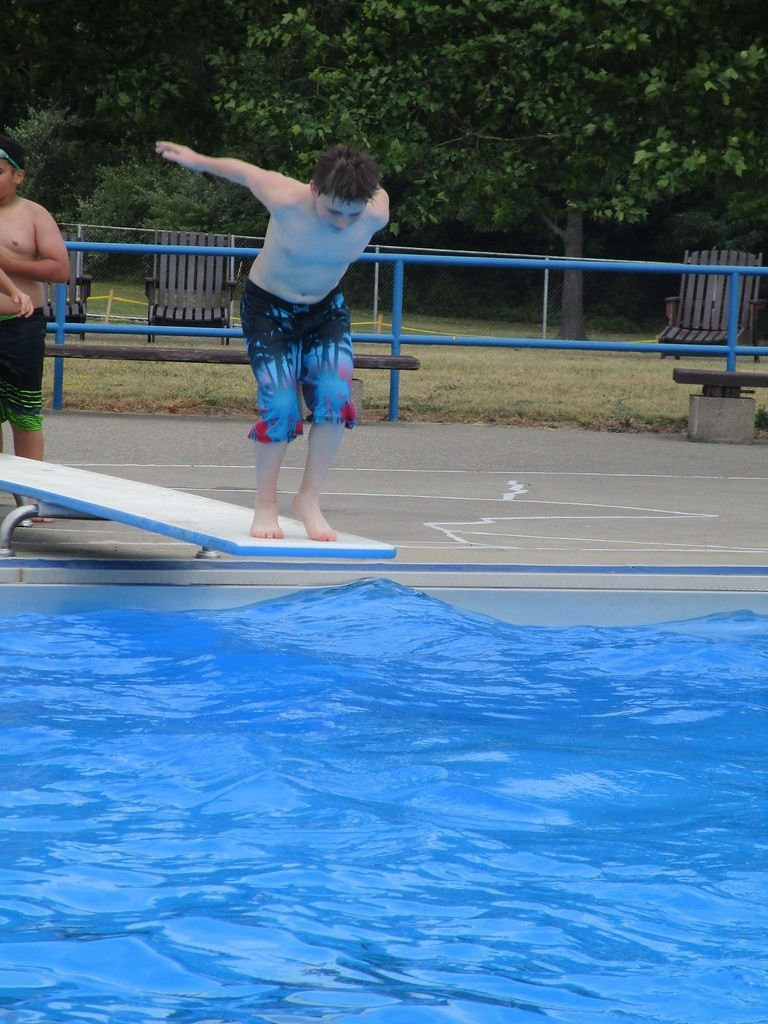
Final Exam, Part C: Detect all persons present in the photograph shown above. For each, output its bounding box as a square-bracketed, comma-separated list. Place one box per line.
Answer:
[154, 140, 389, 541]
[0, 132, 71, 522]
[0, 268, 34, 318]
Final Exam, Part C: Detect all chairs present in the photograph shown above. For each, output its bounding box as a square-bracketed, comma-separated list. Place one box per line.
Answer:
[659, 251, 760, 363]
[42, 225, 92, 341]
[144, 230, 237, 344]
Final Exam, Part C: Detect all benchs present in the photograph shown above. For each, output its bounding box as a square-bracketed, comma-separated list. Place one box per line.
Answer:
[46, 339, 418, 431]
[672, 367, 768, 446]
